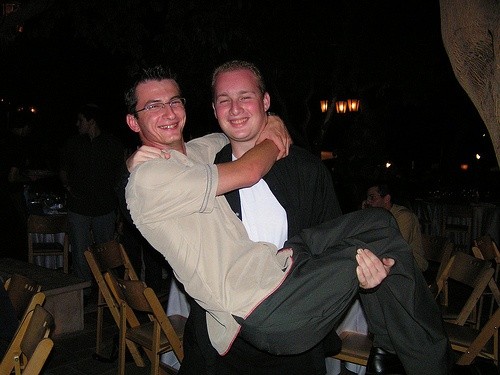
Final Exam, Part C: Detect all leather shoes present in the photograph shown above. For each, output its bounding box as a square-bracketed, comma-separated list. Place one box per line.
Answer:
[365, 345, 406, 375]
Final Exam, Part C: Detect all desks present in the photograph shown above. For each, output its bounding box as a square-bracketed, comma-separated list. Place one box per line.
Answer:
[415, 197, 498, 251]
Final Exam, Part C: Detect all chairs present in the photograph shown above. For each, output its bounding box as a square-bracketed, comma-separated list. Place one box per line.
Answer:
[0, 274, 56, 375]
[28, 215, 73, 273]
[333, 233, 500, 375]
[85, 239, 189, 375]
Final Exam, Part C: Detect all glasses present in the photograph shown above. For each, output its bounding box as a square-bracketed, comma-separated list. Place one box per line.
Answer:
[135, 97, 186, 113]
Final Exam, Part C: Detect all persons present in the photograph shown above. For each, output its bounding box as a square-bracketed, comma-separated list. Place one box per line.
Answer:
[430, 241, 500, 314]
[122, 64, 500, 375]
[117, 59, 396, 375]
[360, 178, 423, 261]
[0, 92, 59, 259]
[57, 103, 124, 308]
